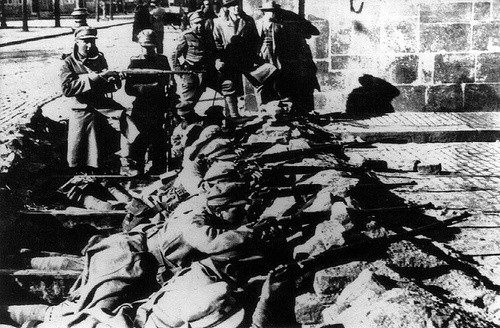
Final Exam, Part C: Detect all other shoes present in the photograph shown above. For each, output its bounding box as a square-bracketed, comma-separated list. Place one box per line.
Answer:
[119, 165, 139, 177]
[66, 186, 83, 204]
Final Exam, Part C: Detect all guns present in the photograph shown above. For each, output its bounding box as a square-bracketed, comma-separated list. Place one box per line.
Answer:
[78, 69, 471, 305]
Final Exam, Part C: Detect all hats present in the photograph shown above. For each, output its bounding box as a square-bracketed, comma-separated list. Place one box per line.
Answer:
[136, 28, 158, 47]
[257, 0, 281, 10]
[198, 124, 223, 144]
[205, 227, 262, 263]
[206, 182, 253, 207]
[187, 10, 207, 23]
[222, 0, 239, 5]
[204, 137, 237, 162]
[203, 159, 239, 185]
[73, 25, 97, 39]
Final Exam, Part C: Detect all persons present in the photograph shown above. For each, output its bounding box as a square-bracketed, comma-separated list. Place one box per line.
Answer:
[0, 96, 292, 327]
[132, 0, 220, 55]
[255, 6, 283, 113]
[125, 29, 176, 179]
[59, 26, 140, 177]
[171, 10, 248, 129]
[212, 0, 278, 113]
